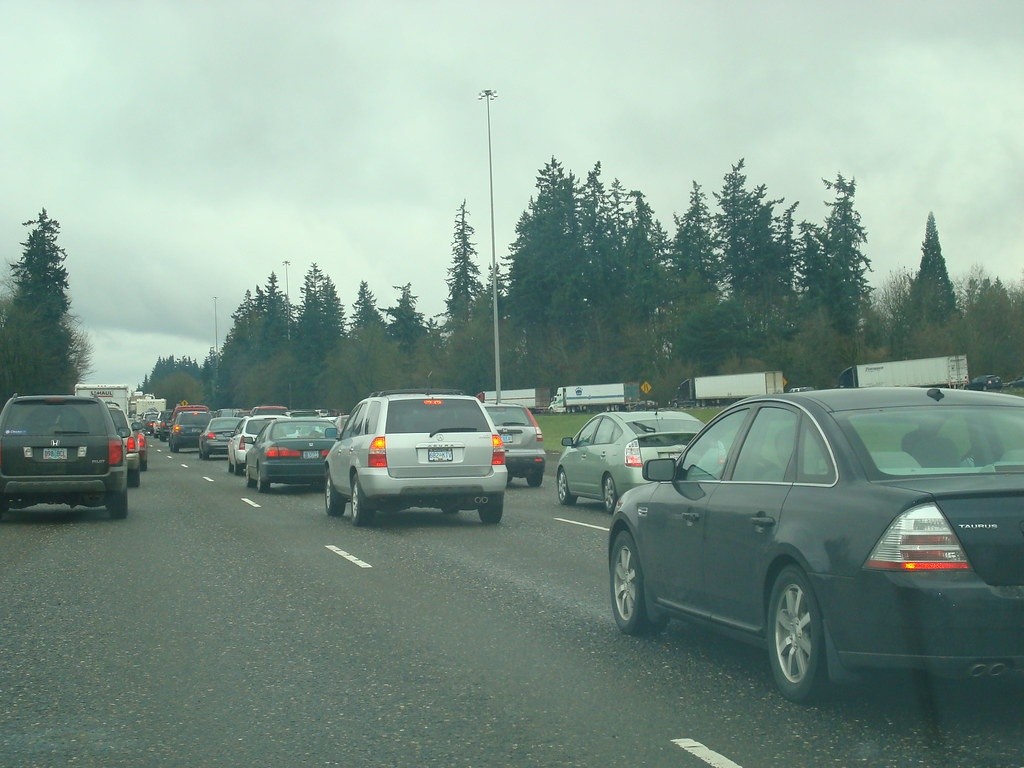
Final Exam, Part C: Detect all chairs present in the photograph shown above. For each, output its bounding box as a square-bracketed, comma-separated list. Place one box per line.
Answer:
[918, 437, 963, 467]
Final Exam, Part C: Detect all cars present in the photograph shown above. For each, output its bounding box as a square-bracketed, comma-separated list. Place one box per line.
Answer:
[969, 375, 1003, 391]
[212, 406, 351, 433]
[199, 417, 242, 460]
[556, 410, 730, 513]
[242, 418, 342, 493]
[129, 417, 148, 470]
[608, 387, 1024, 704]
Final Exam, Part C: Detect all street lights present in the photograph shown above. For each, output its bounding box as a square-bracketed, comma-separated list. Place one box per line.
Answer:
[479, 87, 504, 399]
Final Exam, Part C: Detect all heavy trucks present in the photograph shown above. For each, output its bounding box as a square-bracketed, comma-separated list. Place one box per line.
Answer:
[548, 383, 639, 413]
[476, 388, 551, 414]
[836, 353, 969, 389]
[670, 370, 784, 409]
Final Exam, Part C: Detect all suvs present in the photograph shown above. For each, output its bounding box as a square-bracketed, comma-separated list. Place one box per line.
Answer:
[481, 401, 546, 486]
[106, 401, 144, 487]
[140, 406, 213, 453]
[227, 415, 288, 475]
[324, 390, 508, 528]
[0, 393, 131, 517]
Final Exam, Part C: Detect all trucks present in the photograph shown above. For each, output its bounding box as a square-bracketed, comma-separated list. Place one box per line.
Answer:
[135, 394, 167, 420]
[74, 383, 131, 426]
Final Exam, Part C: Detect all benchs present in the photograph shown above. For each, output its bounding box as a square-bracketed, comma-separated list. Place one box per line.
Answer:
[871, 450, 1024, 466]
